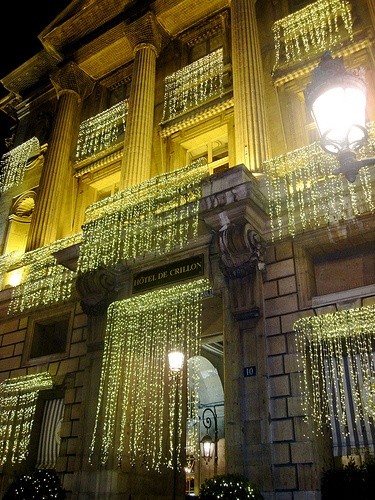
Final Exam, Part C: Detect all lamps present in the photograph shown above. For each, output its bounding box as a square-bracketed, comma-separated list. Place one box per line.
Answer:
[303, 51, 375, 183]
